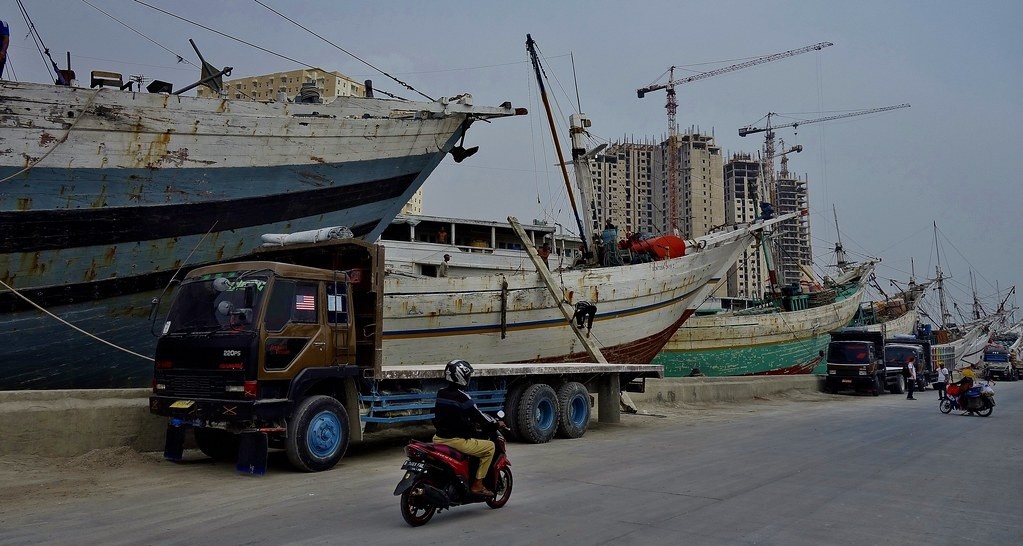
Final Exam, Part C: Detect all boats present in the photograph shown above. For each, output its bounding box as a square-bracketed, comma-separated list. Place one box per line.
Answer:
[0, 21, 528, 391]
[649, 177, 1015, 377]
[382, 201, 806, 394]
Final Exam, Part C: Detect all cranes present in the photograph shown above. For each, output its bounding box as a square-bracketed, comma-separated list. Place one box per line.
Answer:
[739, 104, 911, 218]
[638, 41, 834, 235]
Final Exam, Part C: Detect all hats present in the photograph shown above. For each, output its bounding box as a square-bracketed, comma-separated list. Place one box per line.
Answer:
[606, 219, 611, 222]
[444, 254, 452, 258]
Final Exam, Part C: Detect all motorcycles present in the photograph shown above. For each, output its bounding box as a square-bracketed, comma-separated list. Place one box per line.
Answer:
[940, 383, 996, 417]
[393, 410, 513, 527]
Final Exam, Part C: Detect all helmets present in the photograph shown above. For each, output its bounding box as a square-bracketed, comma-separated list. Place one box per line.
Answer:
[444, 360, 474, 392]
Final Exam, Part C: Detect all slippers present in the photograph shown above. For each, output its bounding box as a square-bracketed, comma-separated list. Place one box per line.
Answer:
[471, 486, 494, 497]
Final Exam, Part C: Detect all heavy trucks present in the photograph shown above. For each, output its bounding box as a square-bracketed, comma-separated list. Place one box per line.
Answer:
[826, 330, 956, 397]
[149, 226, 665, 475]
[984, 343, 1023, 381]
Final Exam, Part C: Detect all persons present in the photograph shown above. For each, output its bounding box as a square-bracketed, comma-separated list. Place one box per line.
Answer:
[438, 252, 450, 278]
[974, 356, 984, 376]
[569, 300, 597, 338]
[951, 369, 976, 416]
[1009, 349, 1017, 377]
[436, 226, 447, 243]
[906, 357, 918, 400]
[0, 19, 10, 79]
[536, 243, 550, 269]
[982, 363, 996, 387]
[604, 219, 614, 229]
[933, 363, 950, 400]
[431, 359, 507, 496]
